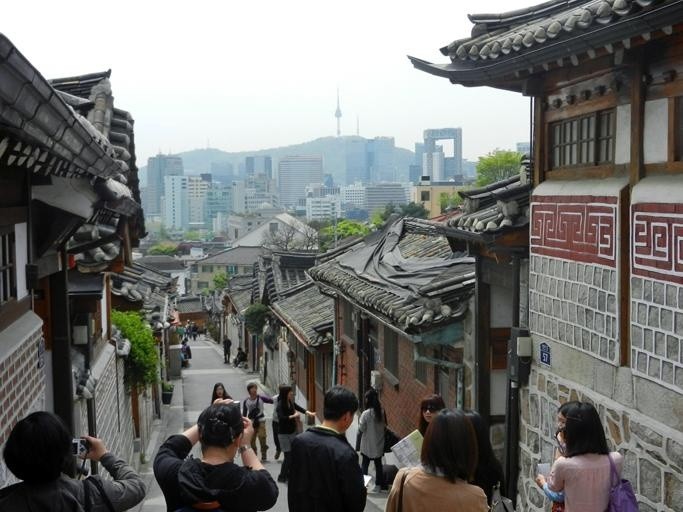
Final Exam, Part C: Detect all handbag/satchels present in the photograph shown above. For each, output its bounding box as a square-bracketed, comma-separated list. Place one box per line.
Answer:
[490, 481, 513, 512]
[248, 407, 265, 428]
[356, 429, 364, 452]
[294, 419, 304, 434]
[607, 452, 640, 512]
[384, 426, 401, 453]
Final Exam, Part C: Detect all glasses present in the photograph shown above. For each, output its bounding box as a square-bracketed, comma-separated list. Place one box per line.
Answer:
[422, 407, 441, 413]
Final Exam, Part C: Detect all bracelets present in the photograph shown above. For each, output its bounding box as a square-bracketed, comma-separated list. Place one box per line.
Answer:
[239, 445, 251, 453]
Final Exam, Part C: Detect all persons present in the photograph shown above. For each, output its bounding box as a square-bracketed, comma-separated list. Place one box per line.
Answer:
[288, 386, 367, 512]
[243, 383, 278, 460]
[277, 386, 316, 482]
[383, 408, 491, 512]
[210, 383, 233, 403]
[548, 402, 623, 512]
[462, 410, 503, 508]
[535, 401, 579, 512]
[359, 390, 387, 493]
[271, 383, 286, 459]
[153, 401, 279, 512]
[418, 394, 445, 438]
[178, 319, 247, 369]
[0, 411, 146, 512]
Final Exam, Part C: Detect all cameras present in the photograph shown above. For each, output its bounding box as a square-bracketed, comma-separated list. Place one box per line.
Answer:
[71, 438, 87, 456]
